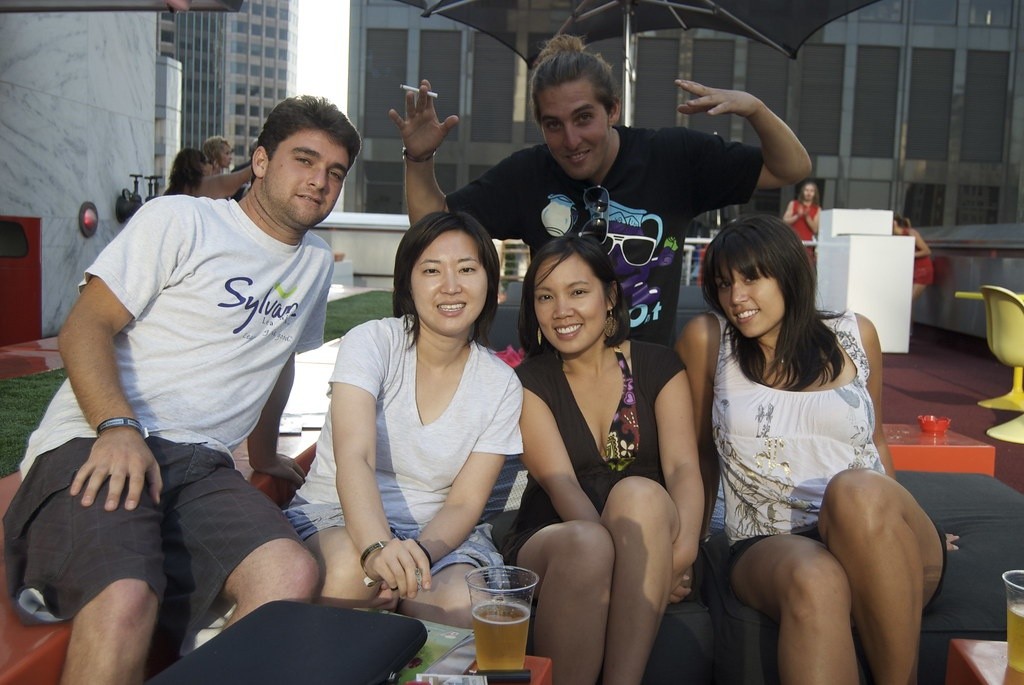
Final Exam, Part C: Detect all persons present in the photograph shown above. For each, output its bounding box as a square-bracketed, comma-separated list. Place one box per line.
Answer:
[163, 136, 258, 202]
[285, 211, 524, 631]
[677, 212, 959, 685]
[491, 236, 706, 685]
[1, 95, 361, 685]
[389, 34, 812, 343]
[893, 213, 934, 338]
[783, 183, 822, 270]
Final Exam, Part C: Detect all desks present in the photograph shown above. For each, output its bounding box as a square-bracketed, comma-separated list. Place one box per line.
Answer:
[954, 290, 1024, 412]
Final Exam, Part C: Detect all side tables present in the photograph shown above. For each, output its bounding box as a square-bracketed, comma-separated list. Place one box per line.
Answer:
[944, 636, 1024, 685]
[882, 424, 996, 479]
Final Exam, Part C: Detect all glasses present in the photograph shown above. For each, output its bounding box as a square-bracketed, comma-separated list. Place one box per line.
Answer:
[579, 186, 609, 245]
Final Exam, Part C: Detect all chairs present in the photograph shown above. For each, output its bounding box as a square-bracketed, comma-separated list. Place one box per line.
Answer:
[980, 284, 1024, 444]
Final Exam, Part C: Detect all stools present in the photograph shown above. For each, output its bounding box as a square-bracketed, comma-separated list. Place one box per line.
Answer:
[493, 510, 710, 685]
[710, 470, 1024, 685]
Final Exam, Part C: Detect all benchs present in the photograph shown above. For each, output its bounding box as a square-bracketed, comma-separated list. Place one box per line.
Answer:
[0, 335, 342, 685]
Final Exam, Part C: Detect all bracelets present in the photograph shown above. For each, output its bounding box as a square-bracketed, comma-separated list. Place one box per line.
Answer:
[403, 147, 437, 162]
[360, 541, 389, 587]
[97, 417, 149, 439]
[415, 539, 432, 569]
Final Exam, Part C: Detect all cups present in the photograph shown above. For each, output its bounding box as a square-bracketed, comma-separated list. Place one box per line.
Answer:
[1001, 570, 1024, 672]
[466, 565, 540, 672]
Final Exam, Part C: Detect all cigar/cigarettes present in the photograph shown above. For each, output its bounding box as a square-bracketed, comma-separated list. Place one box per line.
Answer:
[400, 84, 437, 98]
[416, 569, 422, 591]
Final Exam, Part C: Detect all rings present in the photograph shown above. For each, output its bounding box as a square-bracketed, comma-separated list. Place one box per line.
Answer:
[391, 586, 398, 591]
